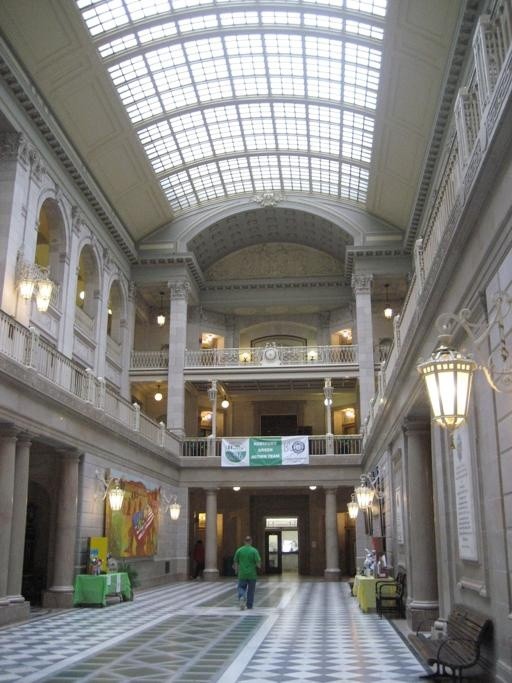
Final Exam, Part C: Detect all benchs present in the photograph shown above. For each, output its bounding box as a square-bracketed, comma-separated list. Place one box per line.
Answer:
[407, 601, 492, 683]
[375, 573, 406, 620]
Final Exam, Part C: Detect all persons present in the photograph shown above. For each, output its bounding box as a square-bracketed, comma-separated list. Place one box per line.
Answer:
[190, 540, 204, 580]
[233, 535, 261, 610]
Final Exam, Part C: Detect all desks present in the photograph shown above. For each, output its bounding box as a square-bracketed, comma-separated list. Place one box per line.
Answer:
[71, 572, 132, 608]
[353, 575, 396, 614]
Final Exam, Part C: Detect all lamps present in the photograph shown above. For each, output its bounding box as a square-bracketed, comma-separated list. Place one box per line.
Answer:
[159, 491, 181, 520]
[156, 291, 166, 328]
[384, 283, 393, 318]
[154, 385, 162, 401]
[309, 486, 316, 490]
[233, 487, 240, 492]
[417, 289, 512, 450]
[347, 493, 360, 519]
[93, 470, 124, 510]
[16, 278, 53, 313]
[222, 396, 229, 408]
[354, 470, 386, 512]
